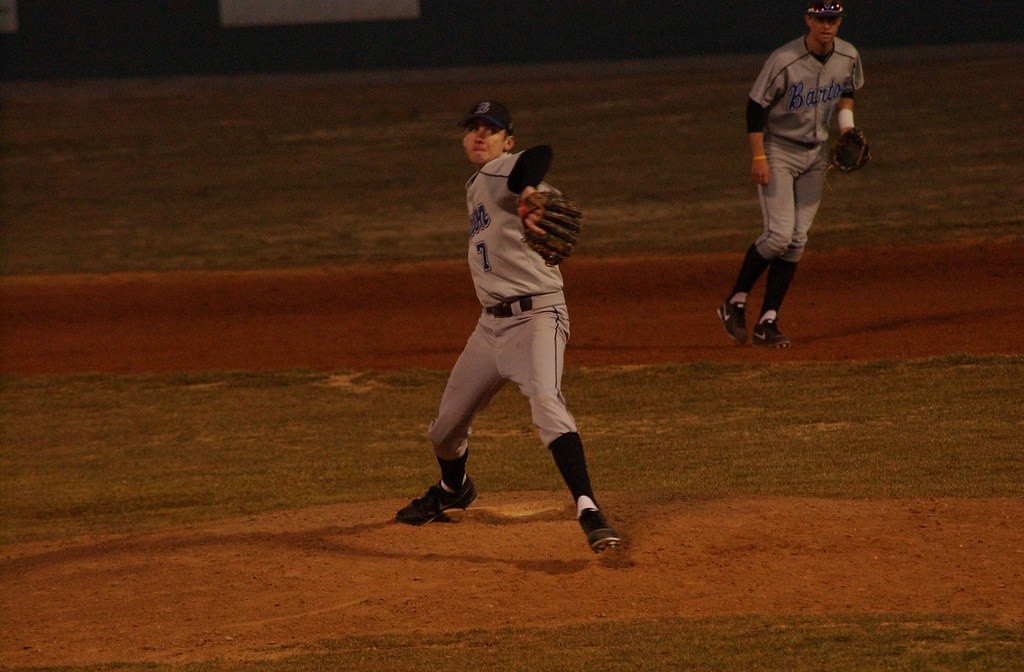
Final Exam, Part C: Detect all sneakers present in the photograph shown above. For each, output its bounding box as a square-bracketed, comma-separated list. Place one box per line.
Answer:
[395, 473, 478, 528]
[752, 318, 791, 349]
[579, 509, 621, 553]
[717, 298, 748, 342]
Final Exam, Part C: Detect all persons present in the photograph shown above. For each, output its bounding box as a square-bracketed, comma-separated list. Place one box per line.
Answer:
[717, 0, 864, 348]
[395, 101, 620, 553]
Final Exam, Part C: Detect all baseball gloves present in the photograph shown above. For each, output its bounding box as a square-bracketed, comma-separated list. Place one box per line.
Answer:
[830, 126, 871, 172]
[517, 189, 585, 267]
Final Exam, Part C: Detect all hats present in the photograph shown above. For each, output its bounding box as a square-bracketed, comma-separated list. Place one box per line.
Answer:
[807, 0, 844, 17]
[459, 99, 514, 136]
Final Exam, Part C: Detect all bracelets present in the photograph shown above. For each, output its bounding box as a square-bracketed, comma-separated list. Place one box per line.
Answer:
[753, 156, 767, 161]
